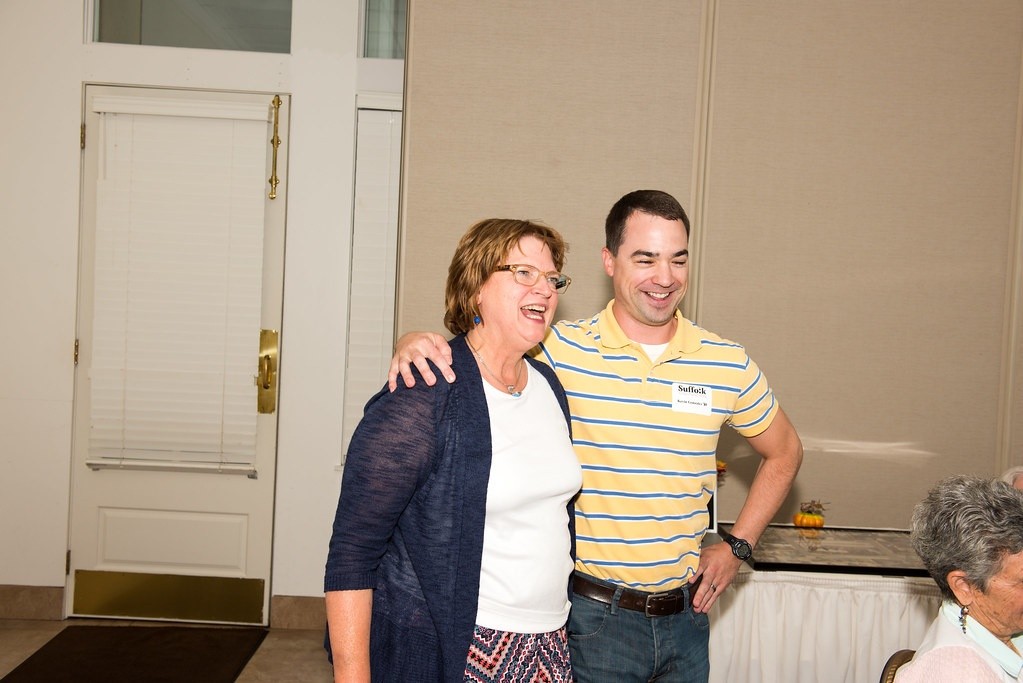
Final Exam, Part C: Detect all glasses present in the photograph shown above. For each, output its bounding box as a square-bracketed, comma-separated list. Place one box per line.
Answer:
[489, 264, 571, 294]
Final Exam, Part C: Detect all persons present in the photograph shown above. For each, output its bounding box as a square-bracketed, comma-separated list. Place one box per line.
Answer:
[892, 475, 1023, 683]
[323, 217, 585, 682]
[386, 189, 803, 683]
[998, 466, 1023, 493]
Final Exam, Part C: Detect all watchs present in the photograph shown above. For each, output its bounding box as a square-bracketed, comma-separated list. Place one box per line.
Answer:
[717, 526, 753, 561]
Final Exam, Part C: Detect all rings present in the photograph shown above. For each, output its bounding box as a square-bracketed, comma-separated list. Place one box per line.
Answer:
[711, 585, 716, 591]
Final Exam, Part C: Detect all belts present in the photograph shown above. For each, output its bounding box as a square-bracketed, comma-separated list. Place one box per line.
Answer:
[570, 572, 701, 618]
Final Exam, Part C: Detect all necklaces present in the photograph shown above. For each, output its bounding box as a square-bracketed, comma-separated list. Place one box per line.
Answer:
[465, 335, 525, 394]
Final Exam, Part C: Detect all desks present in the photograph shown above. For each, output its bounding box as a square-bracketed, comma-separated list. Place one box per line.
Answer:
[708, 523, 946, 683]
